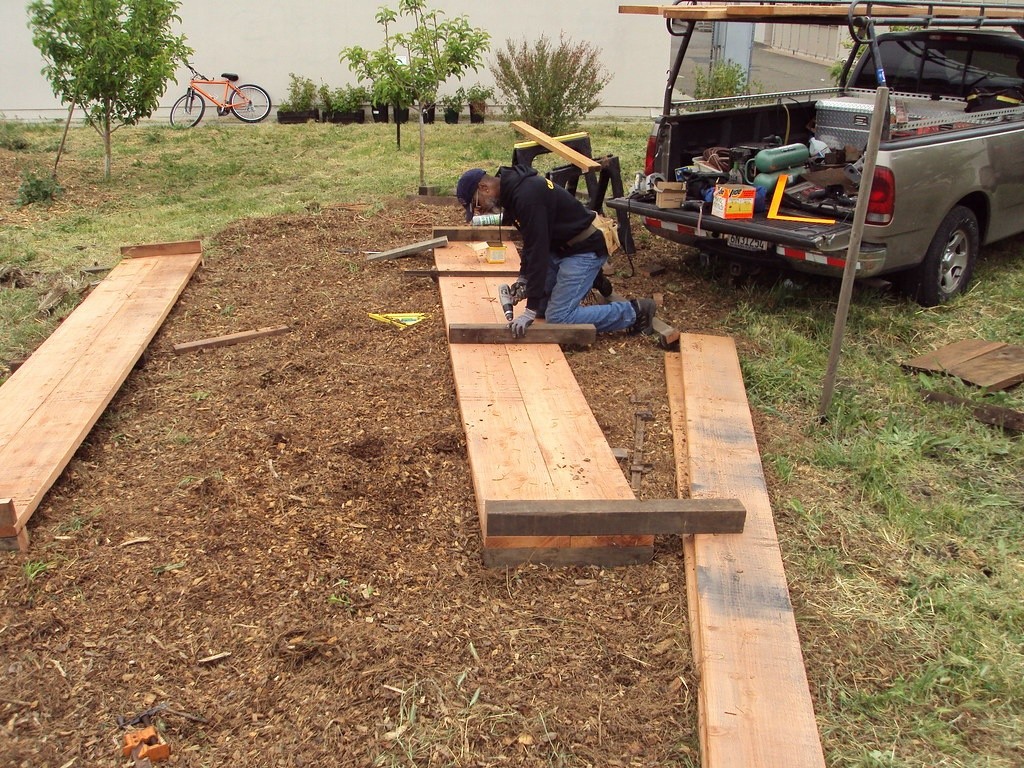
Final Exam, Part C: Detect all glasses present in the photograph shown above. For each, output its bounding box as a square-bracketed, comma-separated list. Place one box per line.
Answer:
[473, 189, 482, 212]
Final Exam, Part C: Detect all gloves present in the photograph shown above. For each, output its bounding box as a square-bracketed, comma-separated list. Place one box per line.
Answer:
[506, 308, 537, 340]
[509, 278, 528, 306]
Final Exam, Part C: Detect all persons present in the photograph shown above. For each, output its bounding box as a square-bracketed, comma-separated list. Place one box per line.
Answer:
[456, 165, 656, 340]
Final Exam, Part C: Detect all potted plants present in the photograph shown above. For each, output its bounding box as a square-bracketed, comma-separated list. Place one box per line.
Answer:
[276, 71, 321, 125]
[416, 88, 438, 125]
[439, 85, 465, 125]
[370, 87, 391, 124]
[319, 78, 368, 124]
[465, 79, 495, 125]
[389, 84, 413, 124]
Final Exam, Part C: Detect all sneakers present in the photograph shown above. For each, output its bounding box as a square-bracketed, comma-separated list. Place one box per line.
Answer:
[629, 296, 657, 334]
[593, 266, 612, 297]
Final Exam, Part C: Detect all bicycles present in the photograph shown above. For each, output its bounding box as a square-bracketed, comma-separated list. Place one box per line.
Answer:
[170, 62, 272, 130]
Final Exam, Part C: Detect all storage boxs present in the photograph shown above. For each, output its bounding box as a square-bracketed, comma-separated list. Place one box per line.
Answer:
[711, 183, 758, 220]
[814, 96, 896, 129]
[811, 126, 869, 154]
[653, 180, 688, 209]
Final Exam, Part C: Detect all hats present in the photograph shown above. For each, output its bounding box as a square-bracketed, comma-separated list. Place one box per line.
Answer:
[456, 169, 486, 223]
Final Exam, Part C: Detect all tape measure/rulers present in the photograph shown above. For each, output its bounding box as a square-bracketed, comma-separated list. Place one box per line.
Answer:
[767, 175, 836, 224]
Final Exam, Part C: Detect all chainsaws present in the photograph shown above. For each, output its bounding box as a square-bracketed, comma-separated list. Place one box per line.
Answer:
[626, 172, 667, 202]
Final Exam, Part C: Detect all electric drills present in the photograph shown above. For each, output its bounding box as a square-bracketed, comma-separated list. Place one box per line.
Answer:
[498, 278, 543, 321]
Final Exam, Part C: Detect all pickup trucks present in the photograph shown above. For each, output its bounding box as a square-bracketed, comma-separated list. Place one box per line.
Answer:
[602, 23, 1024, 308]
